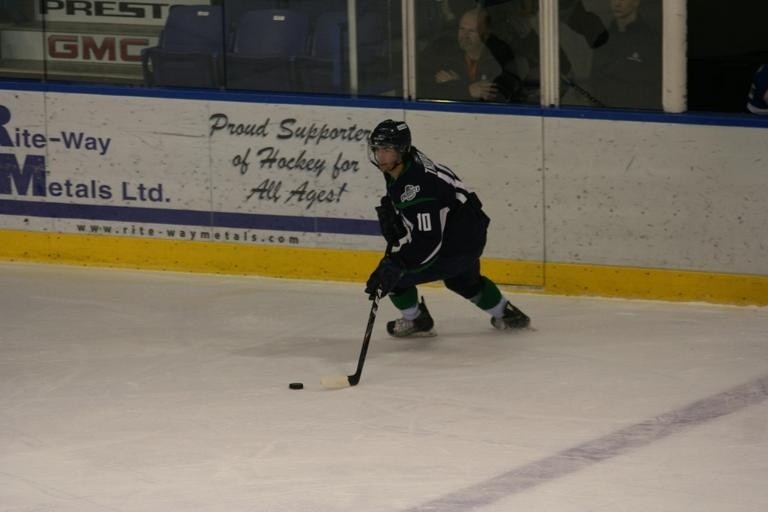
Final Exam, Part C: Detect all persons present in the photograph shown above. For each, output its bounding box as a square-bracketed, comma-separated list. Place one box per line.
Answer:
[364, 119, 531, 338]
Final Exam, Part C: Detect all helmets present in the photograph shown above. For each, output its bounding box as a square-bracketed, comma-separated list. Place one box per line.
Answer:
[364, 117, 412, 149]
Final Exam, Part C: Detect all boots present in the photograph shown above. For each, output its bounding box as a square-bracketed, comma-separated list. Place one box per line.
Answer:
[384, 294, 436, 337]
[489, 300, 533, 330]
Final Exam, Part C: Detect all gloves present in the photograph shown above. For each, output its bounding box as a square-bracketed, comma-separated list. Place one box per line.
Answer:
[361, 261, 397, 299]
[374, 195, 406, 247]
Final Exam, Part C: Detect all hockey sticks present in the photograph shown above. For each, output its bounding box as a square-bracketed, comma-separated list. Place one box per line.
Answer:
[319, 240, 392, 389]
